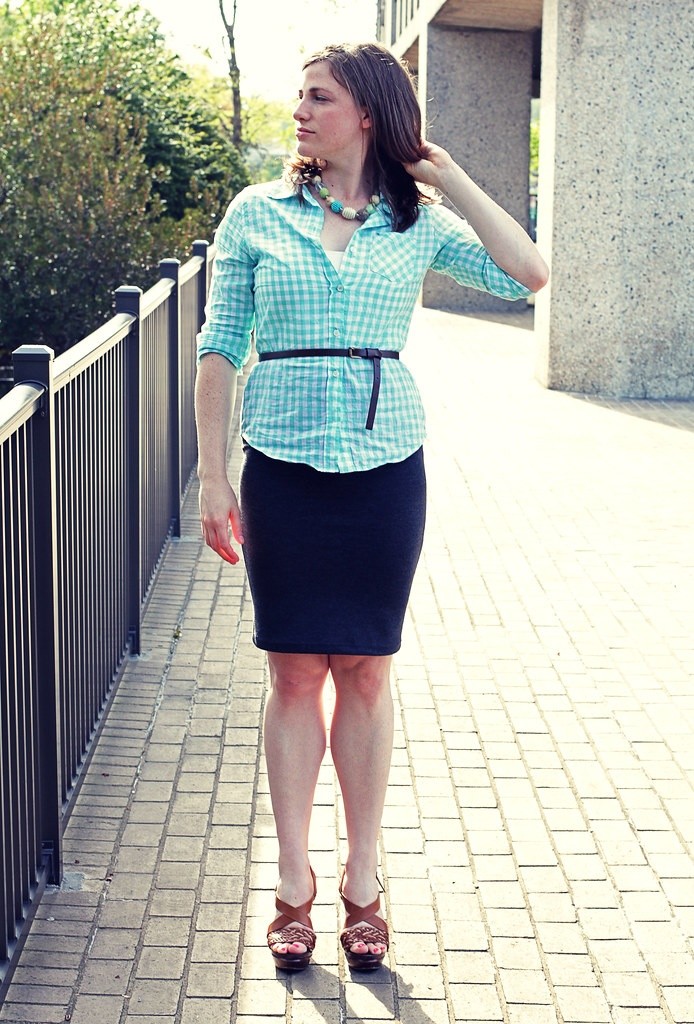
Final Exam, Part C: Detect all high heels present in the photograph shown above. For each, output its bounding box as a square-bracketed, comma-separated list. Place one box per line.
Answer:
[339, 868, 390, 969]
[267, 865, 316, 970]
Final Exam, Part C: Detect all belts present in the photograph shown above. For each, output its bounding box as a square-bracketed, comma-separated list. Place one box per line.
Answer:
[259, 348, 398, 431]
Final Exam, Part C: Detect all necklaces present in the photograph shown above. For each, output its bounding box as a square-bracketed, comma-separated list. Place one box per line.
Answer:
[312, 176, 380, 222]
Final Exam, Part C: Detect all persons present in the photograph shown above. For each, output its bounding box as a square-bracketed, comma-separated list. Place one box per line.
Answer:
[195, 46, 548, 969]
